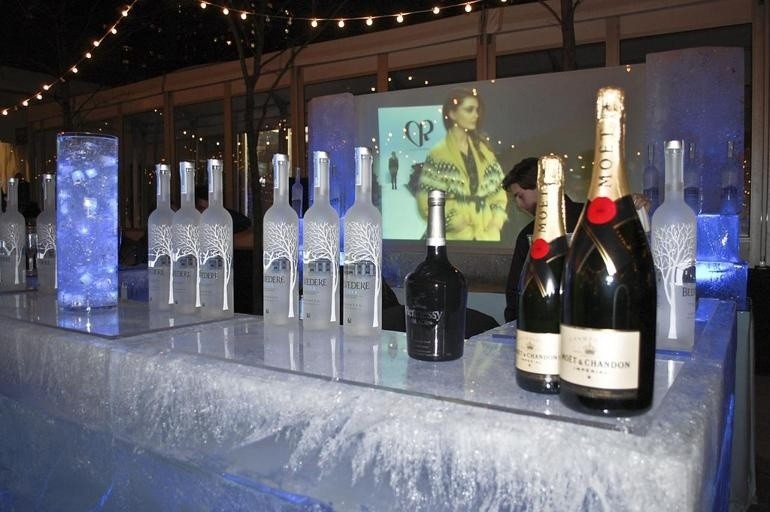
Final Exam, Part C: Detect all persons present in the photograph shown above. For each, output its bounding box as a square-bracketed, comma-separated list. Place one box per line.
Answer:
[3, 172, 32, 220]
[388, 151, 399, 188]
[196, 186, 250, 236]
[501, 156, 653, 322]
[418, 87, 508, 245]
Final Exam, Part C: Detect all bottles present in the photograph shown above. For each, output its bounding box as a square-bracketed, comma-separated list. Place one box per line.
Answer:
[37, 173, 60, 287]
[515, 156, 567, 395]
[0, 177, 28, 291]
[684, 139, 741, 215]
[640, 141, 660, 215]
[342, 150, 382, 337]
[649, 134, 697, 351]
[557, 87, 657, 418]
[264, 149, 298, 325]
[171, 162, 201, 311]
[205, 159, 233, 313]
[26, 224, 37, 277]
[304, 152, 340, 329]
[149, 164, 176, 311]
[403, 192, 466, 362]
